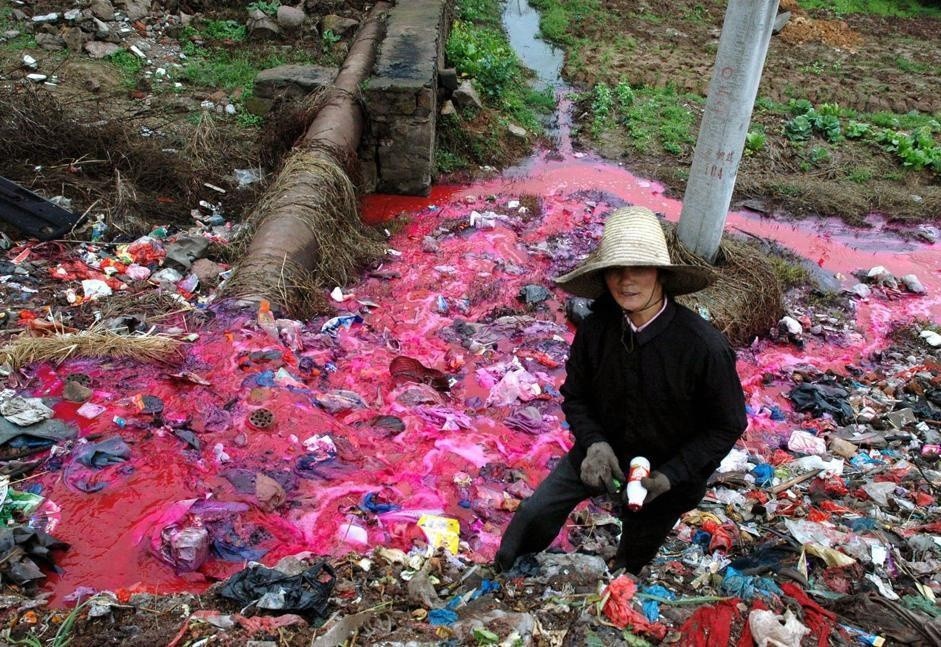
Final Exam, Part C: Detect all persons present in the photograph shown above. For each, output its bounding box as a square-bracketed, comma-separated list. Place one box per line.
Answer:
[495, 204, 750, 586]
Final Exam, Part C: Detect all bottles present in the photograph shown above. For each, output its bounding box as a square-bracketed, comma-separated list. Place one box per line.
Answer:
[626, 456, 651, 512]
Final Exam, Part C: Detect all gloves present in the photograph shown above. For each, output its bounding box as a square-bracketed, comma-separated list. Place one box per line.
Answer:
[622, 470, 672, 506]
[580, 442, 626, 494]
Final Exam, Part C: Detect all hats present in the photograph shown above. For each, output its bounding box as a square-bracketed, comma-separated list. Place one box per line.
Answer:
[556, 206, 718, 299]
